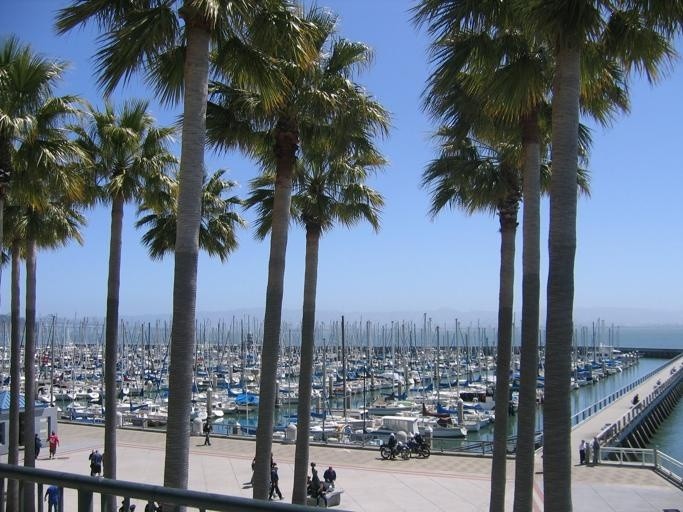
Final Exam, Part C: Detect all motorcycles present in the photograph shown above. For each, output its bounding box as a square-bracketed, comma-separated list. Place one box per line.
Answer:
[380, 441, 431, 460]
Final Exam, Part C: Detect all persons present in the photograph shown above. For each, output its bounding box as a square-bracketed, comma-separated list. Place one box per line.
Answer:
[44, 485, 59, 512]
[578, 439, 587, 465]
[202, 419, 214, 446]
[251, 450, 336, 509]
[591, 436, 601, 466]
[35, 434, 42, 459]
[387, 431, 398, 460]
[656, 376, 663, 388]
[144, 500, 156, 512]
[414, 432, 425, 457]
[155, 501, 162, 512]
[119, 500, 125, 511]
[101, 449, 105, 477]
[129, 504, 136, 512]
[91, 450, 103, 477]
[45, 431, 61, 459]
[632, 393, 642, 409]
[88, 449, 95, 477]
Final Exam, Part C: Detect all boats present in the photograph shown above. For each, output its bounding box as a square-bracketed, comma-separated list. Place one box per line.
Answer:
[1, 309, 640, 442]
